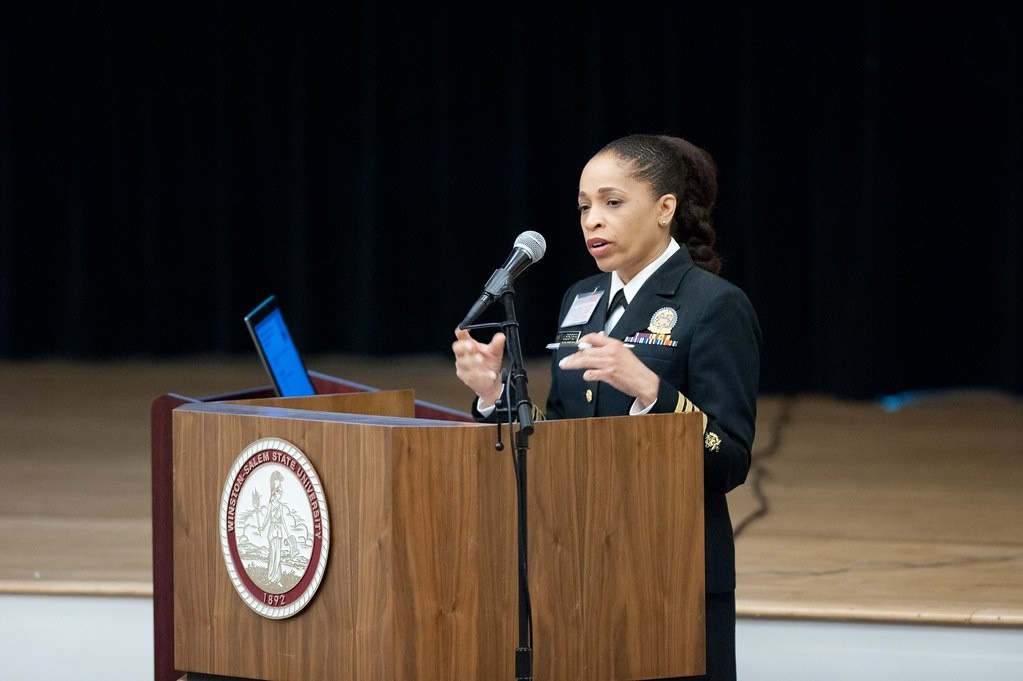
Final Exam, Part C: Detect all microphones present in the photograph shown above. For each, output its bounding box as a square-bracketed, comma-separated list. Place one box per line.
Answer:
[460, 230, 547, 327]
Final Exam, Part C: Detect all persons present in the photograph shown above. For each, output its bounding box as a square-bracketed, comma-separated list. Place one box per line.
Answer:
[452, 134, 762, 681]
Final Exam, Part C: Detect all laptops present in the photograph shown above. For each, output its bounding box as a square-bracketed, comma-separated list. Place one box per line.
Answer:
[244, 295, 317, 398]
